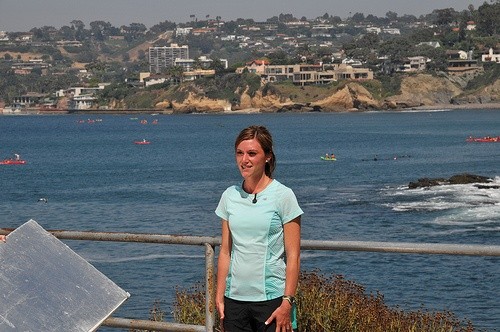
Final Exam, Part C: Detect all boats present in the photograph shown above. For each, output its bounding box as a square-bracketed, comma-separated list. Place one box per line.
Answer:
[135, 141, 150, 144]
[464, 136, 500, 142]
[73, 116, 161, 126]
[1, 160, 28, 165]
[321, 156, 336, 161]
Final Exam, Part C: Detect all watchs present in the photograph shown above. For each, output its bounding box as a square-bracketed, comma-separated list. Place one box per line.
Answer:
[282, 295, 296, 305]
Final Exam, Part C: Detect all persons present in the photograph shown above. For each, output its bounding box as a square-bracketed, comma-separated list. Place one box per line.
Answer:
[214, 124, 303, 332]
[325, 154, 336, 158]
